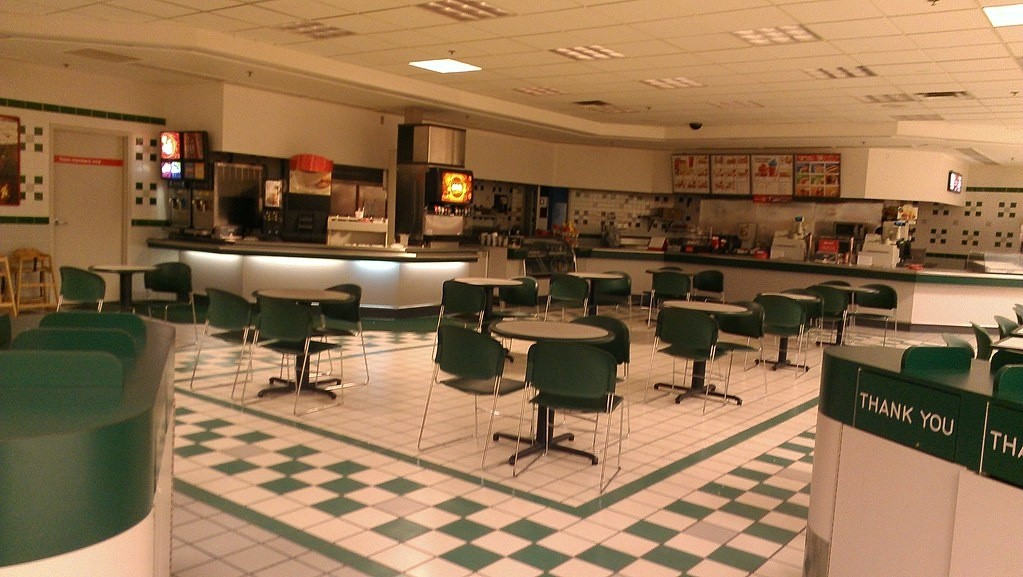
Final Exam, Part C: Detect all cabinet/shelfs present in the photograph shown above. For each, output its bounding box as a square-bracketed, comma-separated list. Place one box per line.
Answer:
[332, 179, 389, 219]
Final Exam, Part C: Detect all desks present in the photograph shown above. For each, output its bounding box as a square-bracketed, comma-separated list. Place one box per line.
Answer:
[646, 268, 697, 324]
[250, 288, 356, 401]
[490, 321, 614, 468]
[753, 292, 820, 372]
[566, 272, 623, 316]
[452, 279, 523, 364]
[990, 335, 1023, 354]
[655, 300, 753, 406]
[815, 283, 880, 349]
[90, 265, 160, 313]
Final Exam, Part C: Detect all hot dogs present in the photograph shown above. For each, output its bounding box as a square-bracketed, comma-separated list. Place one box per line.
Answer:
[826, 165, 839, 173]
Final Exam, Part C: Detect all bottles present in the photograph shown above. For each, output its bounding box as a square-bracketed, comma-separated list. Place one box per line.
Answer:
[757, 159, 777, 176]
[434, 204, 471, 217]
[552, 230, 579, 247]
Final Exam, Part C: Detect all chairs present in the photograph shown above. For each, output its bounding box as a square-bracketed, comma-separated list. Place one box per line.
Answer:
[0, 248, 61, 315]
[943, 302, 1023, 360]
[638, 263, 898, 416]
[56, 266, 106, 312]
[416, 271, 634, 494]
[144, 261, 198, 351]
[191, 284, 370, 415]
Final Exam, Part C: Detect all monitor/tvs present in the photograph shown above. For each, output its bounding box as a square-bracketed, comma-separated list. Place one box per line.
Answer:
[882, 221, 909, 242]
[947, 171, 963, 193]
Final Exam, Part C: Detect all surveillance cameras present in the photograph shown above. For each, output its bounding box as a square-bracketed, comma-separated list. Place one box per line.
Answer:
[690, 123, 702, 130]
